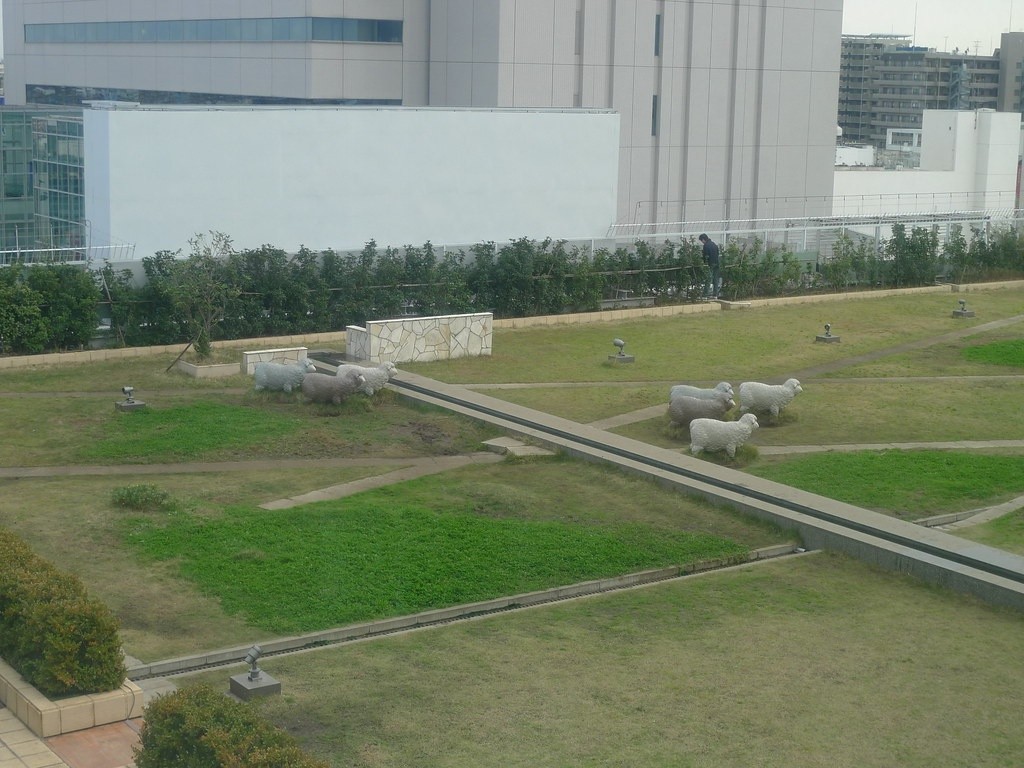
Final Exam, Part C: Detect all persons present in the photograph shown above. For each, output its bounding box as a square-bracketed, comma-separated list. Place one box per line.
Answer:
[699, 234, 720, 298]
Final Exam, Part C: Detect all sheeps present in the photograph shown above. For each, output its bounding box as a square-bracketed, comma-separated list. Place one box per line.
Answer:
[668, 382, 736, 427]
[739, 378, 803, 419]
[255, 358, 398, 410]
[689, 413, 759, 457]
